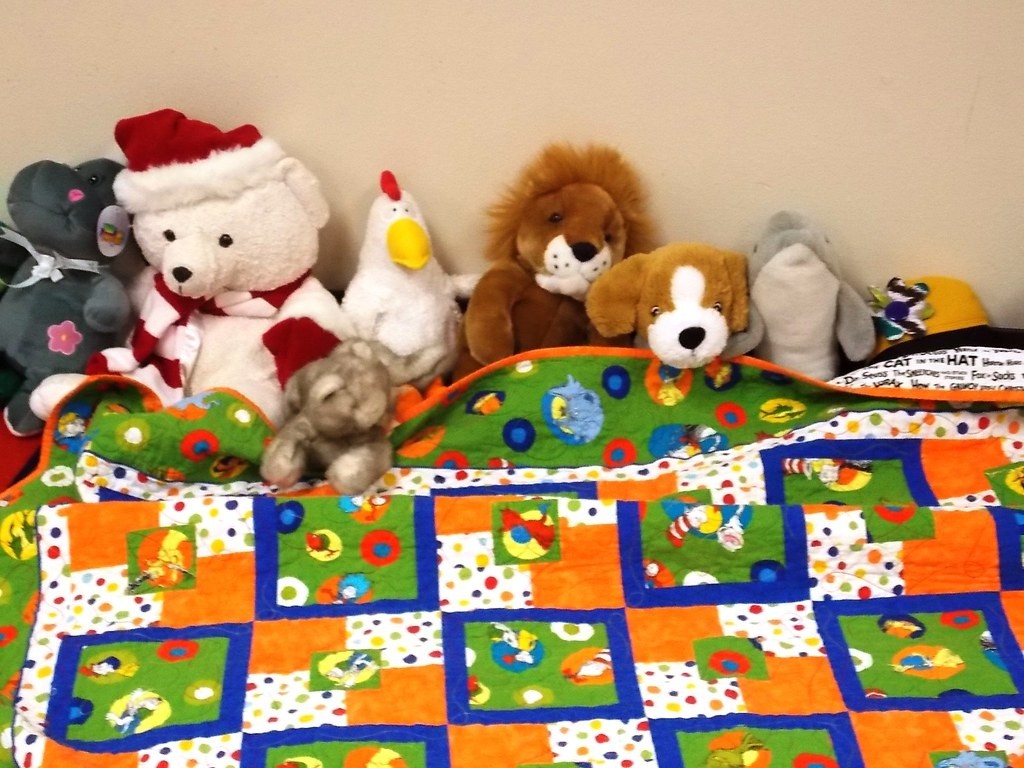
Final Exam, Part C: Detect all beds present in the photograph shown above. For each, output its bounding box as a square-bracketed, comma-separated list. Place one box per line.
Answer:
[0, 324, 1024, 767]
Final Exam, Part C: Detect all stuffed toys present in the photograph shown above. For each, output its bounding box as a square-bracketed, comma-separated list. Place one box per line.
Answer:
[333, 169, 486, 393]
[2, 108, 358, 439]
[724, 207, 875, 385]
[259, 314, 449, 494]
[461, 141, 657, 371]
[0, 158, 150, 402]
[583, 241, 764, 368]
